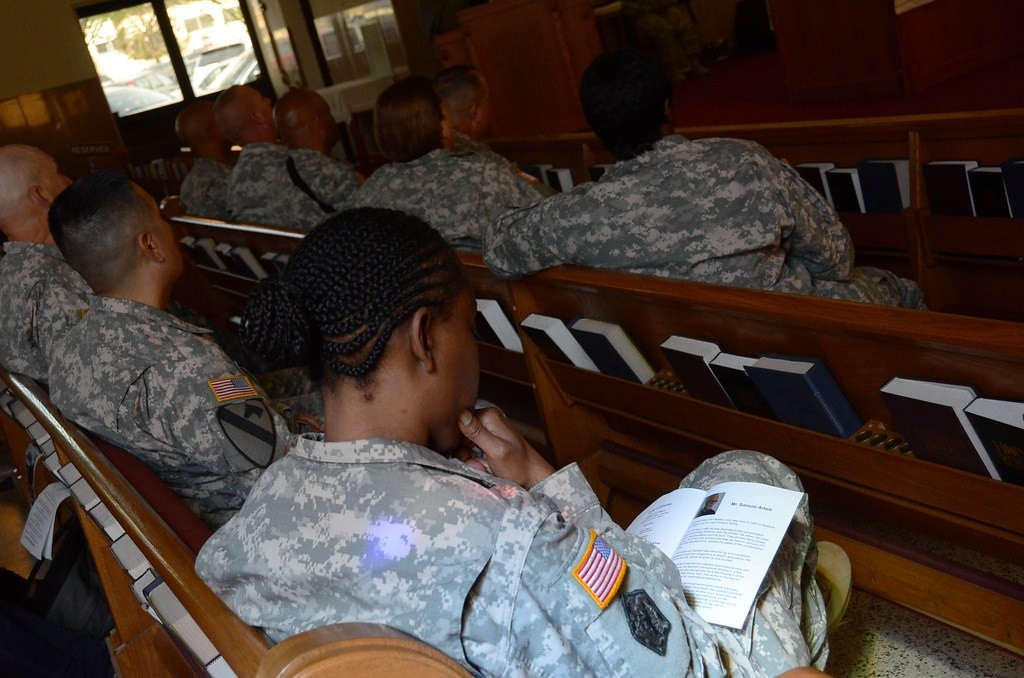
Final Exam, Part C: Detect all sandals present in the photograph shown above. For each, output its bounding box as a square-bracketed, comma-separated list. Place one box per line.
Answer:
[814, 540, 854, 639]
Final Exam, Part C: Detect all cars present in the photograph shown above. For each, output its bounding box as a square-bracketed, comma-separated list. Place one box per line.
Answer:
[91, 13, 402, 120]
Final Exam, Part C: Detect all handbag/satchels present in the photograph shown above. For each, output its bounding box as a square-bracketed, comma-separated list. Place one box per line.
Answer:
[0, 596, 112, 678]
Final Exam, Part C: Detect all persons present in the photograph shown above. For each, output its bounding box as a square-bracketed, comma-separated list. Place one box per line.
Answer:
[352, 63, 547, 258]
[483, 45, 929, 313]
[620, 1, 712, 82]
[1, 142, 74, 385]
[173, 84, 363, 231]
[47, 167, 300, 532]
[192, 207, 854, 678]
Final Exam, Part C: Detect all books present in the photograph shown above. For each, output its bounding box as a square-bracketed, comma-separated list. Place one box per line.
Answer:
[526, 150, 615, 201]
[473, 276, 1022, 500]
[175, 223, 300, 297]
[783, 150, 1023, 225]
[0, 383, 242, 675]
[620, 482, 803, 633]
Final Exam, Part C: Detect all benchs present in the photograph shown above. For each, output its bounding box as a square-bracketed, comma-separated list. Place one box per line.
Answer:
[0, 104, 1024, 678]
